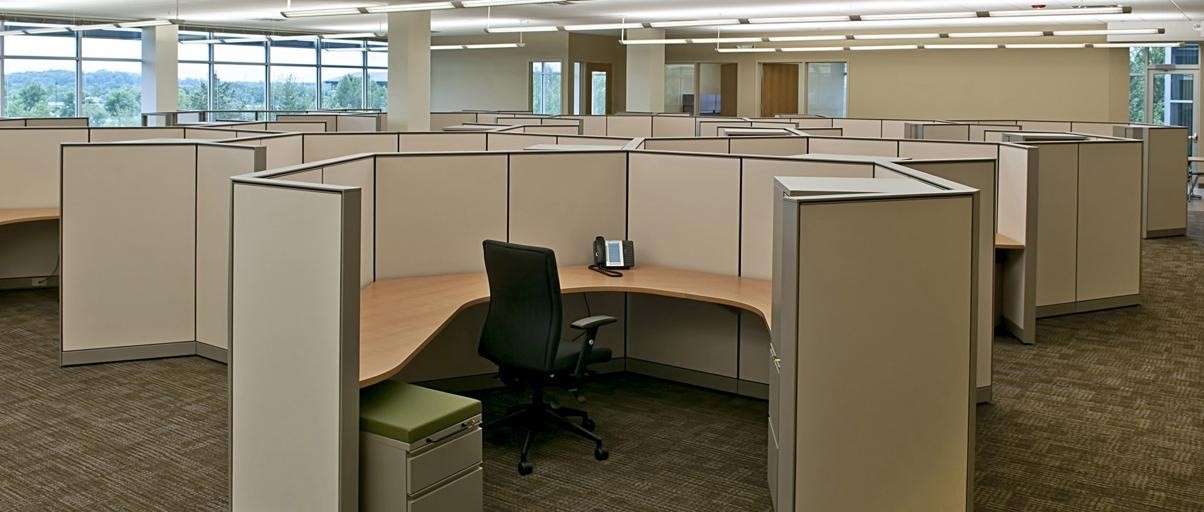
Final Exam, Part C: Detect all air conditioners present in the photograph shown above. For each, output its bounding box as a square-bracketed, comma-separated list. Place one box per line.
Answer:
[1106, 12, 1204, 42]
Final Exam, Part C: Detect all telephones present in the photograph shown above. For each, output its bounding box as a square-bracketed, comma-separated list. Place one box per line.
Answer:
[593, 236, 635, 270]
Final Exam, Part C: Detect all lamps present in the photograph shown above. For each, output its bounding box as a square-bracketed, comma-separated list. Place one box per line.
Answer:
[0, 0, 1185, 53]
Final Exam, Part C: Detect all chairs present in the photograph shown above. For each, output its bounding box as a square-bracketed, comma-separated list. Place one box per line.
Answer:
[468, 239, 617, 475]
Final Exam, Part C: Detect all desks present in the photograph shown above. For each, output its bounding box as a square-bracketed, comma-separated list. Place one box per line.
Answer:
[1187, 156, 1204, 202]
[359, 265, 772, 388]
[995, 233, 1024, 250]
[0, 207, 60, 225]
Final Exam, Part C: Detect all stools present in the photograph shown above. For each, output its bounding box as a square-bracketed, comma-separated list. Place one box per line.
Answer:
[1187, 171, 1204, 200]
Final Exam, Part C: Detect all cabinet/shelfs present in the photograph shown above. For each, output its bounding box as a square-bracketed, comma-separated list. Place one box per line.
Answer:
[360, 380, 483, 512]
